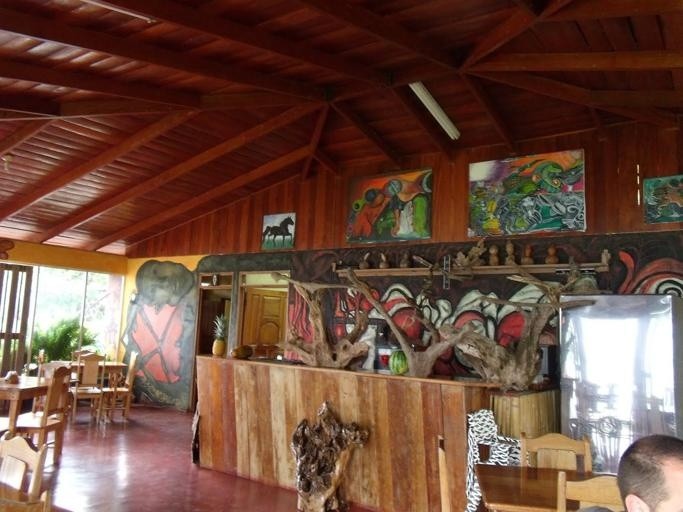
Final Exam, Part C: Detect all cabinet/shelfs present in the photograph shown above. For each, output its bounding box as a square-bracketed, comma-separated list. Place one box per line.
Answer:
[330, 237, 611, 291]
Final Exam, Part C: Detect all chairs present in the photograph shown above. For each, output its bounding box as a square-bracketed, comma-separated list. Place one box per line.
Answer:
[1, 338, 140, 512]
[555, 470, 627, 512]
[519, 431, 595, 476]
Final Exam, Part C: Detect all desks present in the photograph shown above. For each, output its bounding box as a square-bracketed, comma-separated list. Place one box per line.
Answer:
[194, 353, 559, 511]
[473, 463, 596, 512]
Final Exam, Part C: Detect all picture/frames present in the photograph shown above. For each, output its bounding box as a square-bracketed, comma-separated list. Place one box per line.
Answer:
[342, 168, 434, 242]
[260, 212, 299, 253]
[466, 146, 587, 240]
[641, 173, 682, 224]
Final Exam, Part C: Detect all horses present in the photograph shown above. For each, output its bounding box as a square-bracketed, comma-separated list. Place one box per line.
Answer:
[262, 216, 294, 247]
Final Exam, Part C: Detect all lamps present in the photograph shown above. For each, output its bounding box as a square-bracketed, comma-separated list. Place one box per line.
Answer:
[407, 80, 461, 145]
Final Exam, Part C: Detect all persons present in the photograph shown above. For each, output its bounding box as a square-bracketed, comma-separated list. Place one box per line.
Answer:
[618, 433, 683, 512]
